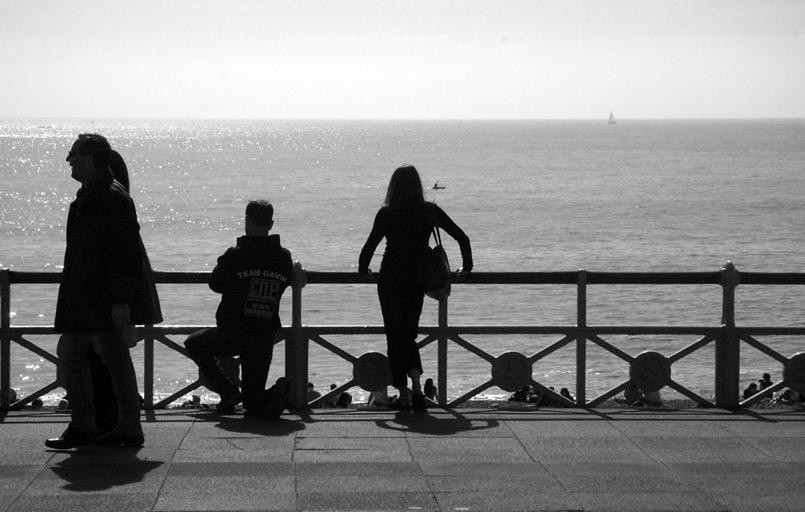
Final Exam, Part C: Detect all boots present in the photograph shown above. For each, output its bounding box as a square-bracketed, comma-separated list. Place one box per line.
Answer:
[94, 390, 144, 447]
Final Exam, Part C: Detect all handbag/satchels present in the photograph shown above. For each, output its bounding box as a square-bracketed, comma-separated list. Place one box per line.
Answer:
[423, 225, 452, 300]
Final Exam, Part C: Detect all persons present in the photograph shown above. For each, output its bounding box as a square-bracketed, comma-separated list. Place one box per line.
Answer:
[308, 382, 352, 408]
[184, 200, 294, 421]
[743, 373, 773, 399]
[44, 134, 163, 449]
[425, 377, 438, 400]
[358, 166, 473, 413]
[509, 385, 576, 403]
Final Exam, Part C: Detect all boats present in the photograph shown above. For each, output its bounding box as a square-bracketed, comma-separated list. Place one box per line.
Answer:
[432, 186, 445, 189]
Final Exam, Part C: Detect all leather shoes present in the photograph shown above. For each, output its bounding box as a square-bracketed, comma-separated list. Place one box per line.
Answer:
[45, 435, 89, 448]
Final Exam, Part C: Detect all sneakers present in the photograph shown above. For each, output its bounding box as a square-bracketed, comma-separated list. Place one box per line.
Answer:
[216, 393, 241, 408]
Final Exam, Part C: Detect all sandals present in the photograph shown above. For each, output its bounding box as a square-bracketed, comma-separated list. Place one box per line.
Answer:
[391, 391, 427, 410]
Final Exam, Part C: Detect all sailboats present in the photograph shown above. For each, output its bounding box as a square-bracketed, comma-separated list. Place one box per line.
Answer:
[607, 111, 616, 124]
